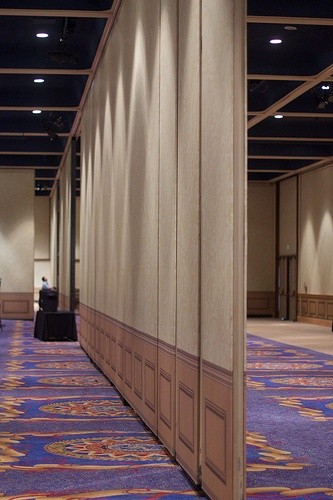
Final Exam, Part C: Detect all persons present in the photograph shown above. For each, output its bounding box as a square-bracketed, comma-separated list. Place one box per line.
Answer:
[40, 276, 56, 292]
